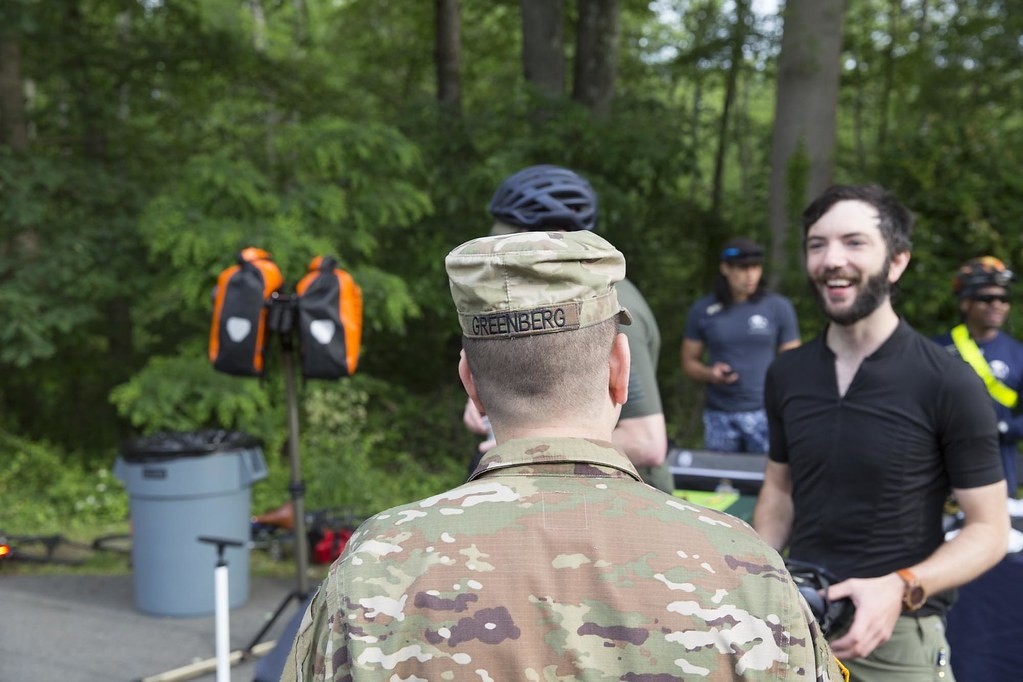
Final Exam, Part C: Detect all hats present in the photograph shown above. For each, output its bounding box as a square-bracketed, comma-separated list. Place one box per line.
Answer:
[445, 231, 626, 339]
[721, 236, 761, 266]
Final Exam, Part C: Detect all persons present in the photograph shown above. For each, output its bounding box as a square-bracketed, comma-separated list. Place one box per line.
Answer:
[458, 164, 673, 493]
[281, 230, 849, 682]
[927, 256, 1023, 503]
[680, 238, 802, 456]
[753, 184, 1010, 682]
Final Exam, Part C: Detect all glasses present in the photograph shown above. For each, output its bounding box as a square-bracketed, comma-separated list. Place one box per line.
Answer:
[722, 246, 765, 260]
[972, 294, 1009, 303]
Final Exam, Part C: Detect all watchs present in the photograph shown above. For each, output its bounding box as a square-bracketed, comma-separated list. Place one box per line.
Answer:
[897, 568, 927, 613]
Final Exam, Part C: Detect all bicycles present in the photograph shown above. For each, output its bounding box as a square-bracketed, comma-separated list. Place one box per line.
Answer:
[89, 503, 384, 553]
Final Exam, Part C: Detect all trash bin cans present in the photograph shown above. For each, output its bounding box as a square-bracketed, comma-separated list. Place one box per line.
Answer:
[113, 430, 270, 615]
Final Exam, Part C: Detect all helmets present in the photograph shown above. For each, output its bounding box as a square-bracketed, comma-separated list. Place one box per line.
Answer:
[489, 165, 598, 230]
[952, 256, 1016, 293]
[783, 558, 847, 636]
